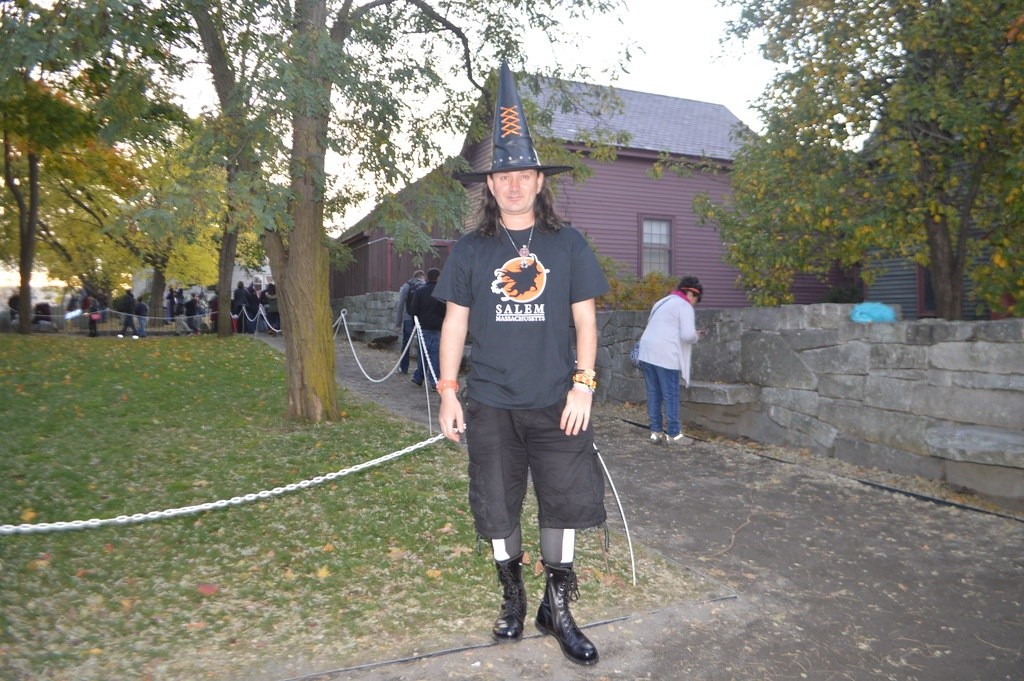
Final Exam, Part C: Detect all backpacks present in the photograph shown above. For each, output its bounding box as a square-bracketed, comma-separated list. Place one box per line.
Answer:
[405, 280, 426, 316]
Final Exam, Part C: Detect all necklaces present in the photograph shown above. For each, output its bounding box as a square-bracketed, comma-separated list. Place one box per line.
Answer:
[500, 219, 535, 269]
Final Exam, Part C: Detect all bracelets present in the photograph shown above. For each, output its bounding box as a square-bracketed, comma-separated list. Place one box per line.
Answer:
[437, 378, 459, 394]
[572, 368, 597, 395]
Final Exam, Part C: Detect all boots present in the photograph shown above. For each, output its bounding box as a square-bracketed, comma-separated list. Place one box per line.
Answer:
[535, 559, 599, 666]
[492, 550, 527, 643]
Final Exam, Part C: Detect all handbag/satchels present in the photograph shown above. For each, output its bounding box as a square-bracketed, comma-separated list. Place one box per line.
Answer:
[91, 313, 102, 321]
[629, 340, 641, 370]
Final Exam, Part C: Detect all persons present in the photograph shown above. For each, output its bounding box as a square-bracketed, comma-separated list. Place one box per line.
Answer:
[7, 289, 20, 322]
[430, 165, 611, 665]
[208, 287, 219, 332]
[165, 288, 208, 336]
[119, 290, 139, 340]
[84, 298, 98, 337]
[639, 276, 706, 445]
[394, 270, 426, 375]
[134, 297, 149, 338]
[410, 268, 447, 392]
[232, 281, 279, 337]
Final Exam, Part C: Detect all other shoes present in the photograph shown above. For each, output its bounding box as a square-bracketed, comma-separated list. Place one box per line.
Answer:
[132, 335, 139, 339]
[664, 433, 694, 447]
[117, 334, 123, 338]
[431, 384, 437, 392]
[650, 431, 668, 444]
[410, 376, 422, 386]
[397, 367, 408, 375]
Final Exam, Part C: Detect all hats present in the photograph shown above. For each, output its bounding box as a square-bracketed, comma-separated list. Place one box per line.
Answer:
[451, 57, 575, 195]
